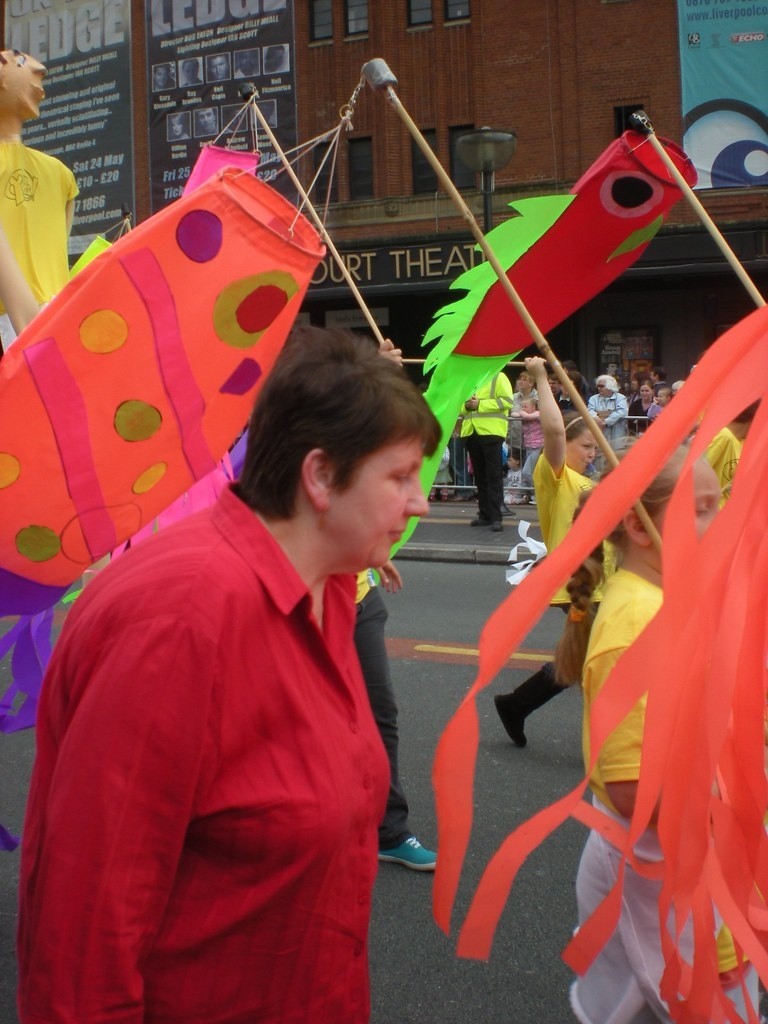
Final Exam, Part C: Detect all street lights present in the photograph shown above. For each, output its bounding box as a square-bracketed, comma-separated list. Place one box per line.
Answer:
[458, 125, 520, 262]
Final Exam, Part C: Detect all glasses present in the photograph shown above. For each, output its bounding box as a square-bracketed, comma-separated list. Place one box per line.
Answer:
[597, 384, 606, 388]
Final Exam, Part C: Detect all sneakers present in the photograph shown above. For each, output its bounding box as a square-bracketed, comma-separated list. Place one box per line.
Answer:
[376, 834, 438, 870]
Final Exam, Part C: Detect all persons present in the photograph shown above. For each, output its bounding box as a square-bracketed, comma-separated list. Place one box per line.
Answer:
[14, 324, 446, 1024]
[153, 45, 286, 141]
[0, 46, 768, 1024]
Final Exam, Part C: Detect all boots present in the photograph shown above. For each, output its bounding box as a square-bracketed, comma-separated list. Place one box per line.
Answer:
[494, 662, 570, 748]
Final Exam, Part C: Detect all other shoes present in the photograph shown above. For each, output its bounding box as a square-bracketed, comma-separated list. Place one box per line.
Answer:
[430, 495, 436, 502]
[442, 495, 448, 501]
[471, 518, 492, 526]
[529, 501, 537, 505]
[493, 520, 503, 532]
